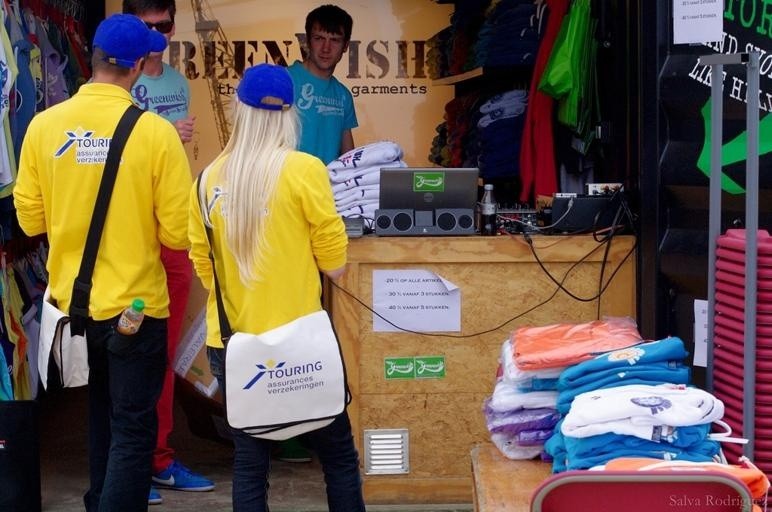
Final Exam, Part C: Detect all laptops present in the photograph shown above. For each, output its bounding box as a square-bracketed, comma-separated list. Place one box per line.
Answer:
[379, 167, 480, 213]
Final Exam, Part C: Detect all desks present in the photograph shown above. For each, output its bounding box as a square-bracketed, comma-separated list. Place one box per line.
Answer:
[467, 429, 552, 512]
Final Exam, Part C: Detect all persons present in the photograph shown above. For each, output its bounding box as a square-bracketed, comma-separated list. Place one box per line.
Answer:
[188, 64, 366, 511]
[121, 0, 215, 503]
[13, 13, 192, 512]
[282, 4, 358, 166]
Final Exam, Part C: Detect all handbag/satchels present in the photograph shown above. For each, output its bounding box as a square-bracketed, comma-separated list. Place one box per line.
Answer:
[38, 284, 91, 392]
[224, 309, 351, 442]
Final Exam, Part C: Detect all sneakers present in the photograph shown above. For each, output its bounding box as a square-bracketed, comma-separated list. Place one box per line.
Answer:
[149, 461, 216, 504]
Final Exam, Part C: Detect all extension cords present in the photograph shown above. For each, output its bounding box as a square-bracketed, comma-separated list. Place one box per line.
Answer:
[583, 183, 625, 197]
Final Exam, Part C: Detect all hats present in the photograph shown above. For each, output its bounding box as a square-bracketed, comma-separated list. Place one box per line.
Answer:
[237, 62, 294, 112]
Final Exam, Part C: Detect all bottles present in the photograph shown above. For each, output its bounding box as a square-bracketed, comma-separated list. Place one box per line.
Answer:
[480, 183, 496, 234]
[116, 299, 145, 338]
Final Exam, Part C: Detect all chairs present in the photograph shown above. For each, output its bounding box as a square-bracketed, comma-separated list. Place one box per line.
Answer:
[529, 470, 753, 512]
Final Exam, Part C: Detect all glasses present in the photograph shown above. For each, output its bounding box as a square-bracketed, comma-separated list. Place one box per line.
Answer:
[142, 21, 175, 34]
[92, 14, 167, 68]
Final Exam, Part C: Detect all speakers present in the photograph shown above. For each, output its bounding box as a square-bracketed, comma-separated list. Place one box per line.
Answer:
[374, 207, 475, 237]
